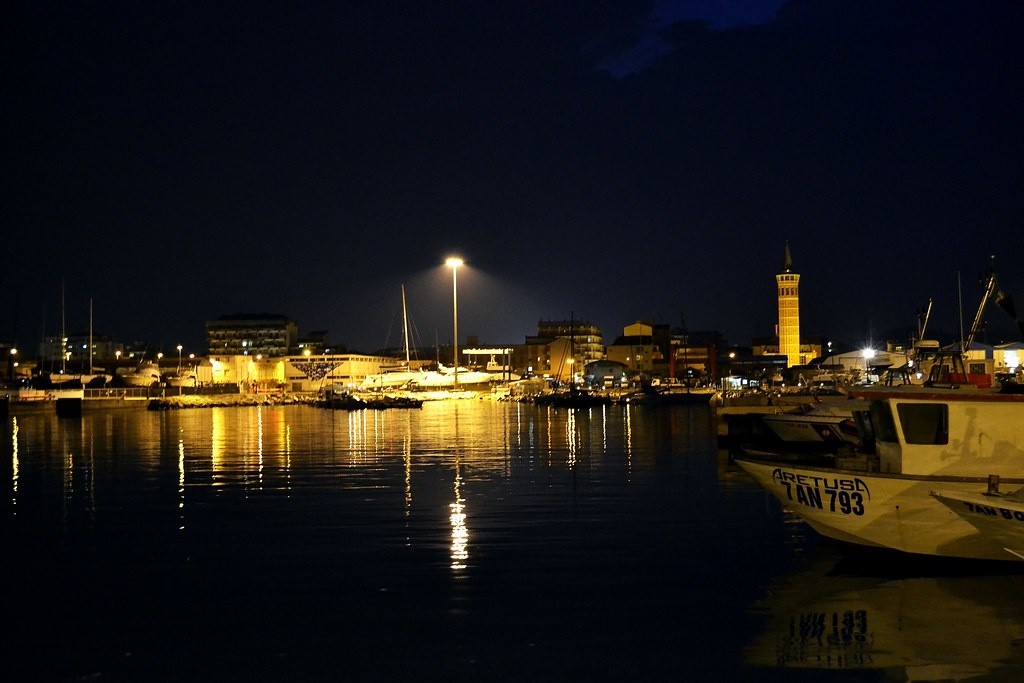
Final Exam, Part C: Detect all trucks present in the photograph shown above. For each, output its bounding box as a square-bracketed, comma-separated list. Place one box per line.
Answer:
[604, 375, 615, 388]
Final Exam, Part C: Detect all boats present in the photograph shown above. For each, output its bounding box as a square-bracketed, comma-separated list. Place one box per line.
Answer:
[715, 270, 1024, 577]
[119, 343, 199, 389]
[736, 557, 1024, 683]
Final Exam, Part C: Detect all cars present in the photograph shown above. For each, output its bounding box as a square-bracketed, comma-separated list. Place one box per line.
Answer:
[615, 382, 619, 388]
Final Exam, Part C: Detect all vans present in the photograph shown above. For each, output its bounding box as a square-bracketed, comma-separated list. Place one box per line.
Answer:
[621, 381, 628, 388]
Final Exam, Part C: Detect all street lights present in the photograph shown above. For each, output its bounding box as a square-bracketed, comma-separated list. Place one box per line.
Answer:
[443, 253, 463, 387]
[176, 343, 183, 395]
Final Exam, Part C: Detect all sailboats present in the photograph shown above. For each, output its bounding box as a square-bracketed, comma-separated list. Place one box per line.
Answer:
[363, 284, 493, 388]
[40, 282, 114, 389]
[535, 311, 611, 408]
